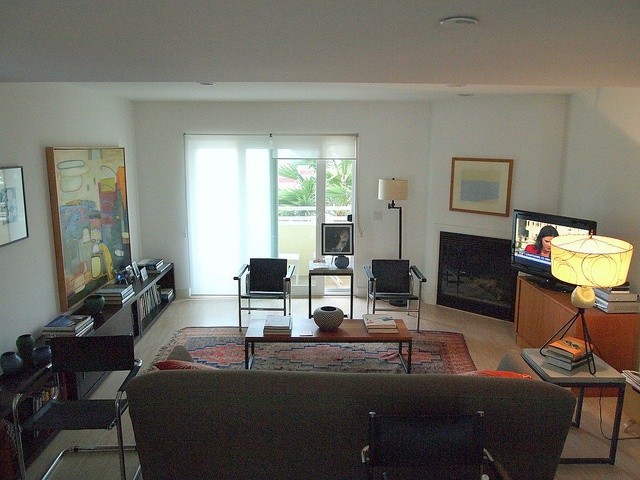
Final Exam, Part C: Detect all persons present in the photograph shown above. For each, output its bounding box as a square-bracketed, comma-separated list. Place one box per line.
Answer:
[326, 228, 349, 252]
[523, 225, 559, 262]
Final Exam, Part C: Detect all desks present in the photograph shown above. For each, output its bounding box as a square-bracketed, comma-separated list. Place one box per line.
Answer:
[514, 277, 639, 398]
[279, 254, 299, 285]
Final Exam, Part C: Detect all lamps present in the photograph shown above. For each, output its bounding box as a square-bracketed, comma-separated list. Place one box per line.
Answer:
[378, 178, 409, 307]
[540, 231, 633, 375]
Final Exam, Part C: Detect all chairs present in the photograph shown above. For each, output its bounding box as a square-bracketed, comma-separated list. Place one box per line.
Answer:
[365, 260, 426, 333]
[233, 258, 295, 332]
[361, 413, 509, 480]
[12, 336, 143, 480]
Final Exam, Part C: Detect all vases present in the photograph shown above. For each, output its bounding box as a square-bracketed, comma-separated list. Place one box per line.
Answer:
[85, 295, 105, 314]
[1, 352, 24, 379]
[34, 345, 52, 365]
[17, 334, 34, 361]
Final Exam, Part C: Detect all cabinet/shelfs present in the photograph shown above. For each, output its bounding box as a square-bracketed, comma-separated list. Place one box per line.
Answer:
[138, 263, 177, 336]
[73, 300, 138, 401]
[0, 364, 66, 480]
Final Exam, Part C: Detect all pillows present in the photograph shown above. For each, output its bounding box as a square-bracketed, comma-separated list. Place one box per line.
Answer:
[458, 371, 532, 379]
[153, 361, 219, 371]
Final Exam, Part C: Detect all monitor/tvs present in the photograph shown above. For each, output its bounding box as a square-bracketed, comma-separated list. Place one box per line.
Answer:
[511, 209, 597, 293]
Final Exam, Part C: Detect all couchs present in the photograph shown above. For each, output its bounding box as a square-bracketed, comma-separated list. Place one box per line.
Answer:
[127, 344, 576, 479]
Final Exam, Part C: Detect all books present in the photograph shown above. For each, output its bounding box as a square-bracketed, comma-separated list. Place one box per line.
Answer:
[137, 259, 170, 274]
[543, 335, 594, 372]
[22, 384, 62, 438]
[92, 284, 136, 305]
[139, 282, 174, 323]
[620, 369, 640, 392]
[313, 262, 329, 270]
[366, 325, 400, 334]
[362, 314, 397, 329]
[41, 312, 95, 344]
[262, 314, 294, 335]
[593, 281, 640, 314]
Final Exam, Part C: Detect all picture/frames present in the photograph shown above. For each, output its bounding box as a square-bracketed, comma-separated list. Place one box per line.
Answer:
[0, 167, 28, 247]
[46, 146, 132, 311]
[321, 223, 354, 256]
[448, 158, 514, 216]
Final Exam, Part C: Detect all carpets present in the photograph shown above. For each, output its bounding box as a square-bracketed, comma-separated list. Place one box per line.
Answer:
[144, 326, 478, 373]
